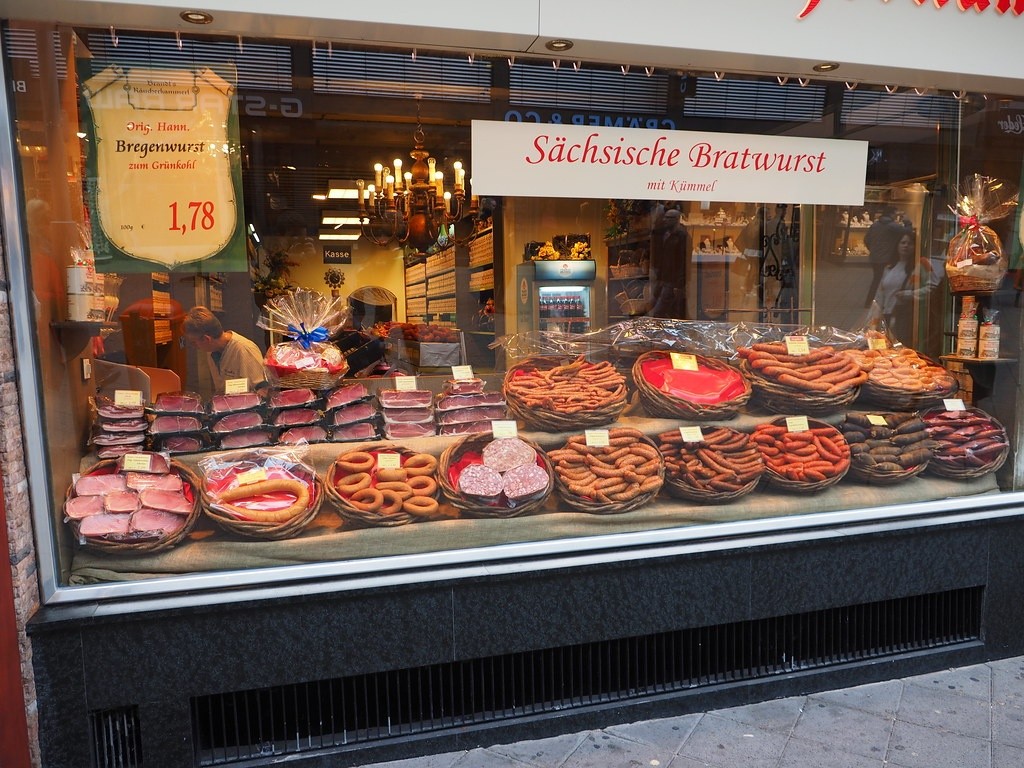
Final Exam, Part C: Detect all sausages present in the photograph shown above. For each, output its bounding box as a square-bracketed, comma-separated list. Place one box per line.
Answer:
[507, 351, 663, 504]
[218, 479, 310, 522]
[334, 451, 438, 515]
[657, 338, 955, 492]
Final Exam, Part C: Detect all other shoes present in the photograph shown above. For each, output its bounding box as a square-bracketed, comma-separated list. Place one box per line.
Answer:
[866, 300, 873, 308]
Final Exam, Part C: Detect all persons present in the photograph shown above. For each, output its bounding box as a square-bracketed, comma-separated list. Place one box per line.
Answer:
[180, 306, 268, 394]
[645, 199, 939, 342]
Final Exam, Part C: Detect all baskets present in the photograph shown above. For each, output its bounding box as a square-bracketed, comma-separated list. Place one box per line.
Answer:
[55, 340, 1012, 558]
[943, 259, 1007, 291]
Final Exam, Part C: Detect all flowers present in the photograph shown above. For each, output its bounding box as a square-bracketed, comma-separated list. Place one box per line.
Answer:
[566, 241, 592, 261]
[603, 197, 653, 239]
[528, 239, 560, 261]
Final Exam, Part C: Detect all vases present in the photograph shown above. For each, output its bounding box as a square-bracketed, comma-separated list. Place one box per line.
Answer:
[625, 213, 655, 242]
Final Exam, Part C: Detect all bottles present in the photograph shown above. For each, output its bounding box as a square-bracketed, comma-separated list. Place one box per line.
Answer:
[537, 294, 588, 333]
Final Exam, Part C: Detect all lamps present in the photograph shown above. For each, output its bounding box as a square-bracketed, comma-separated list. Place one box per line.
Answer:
[352, 92, 489, 266]
